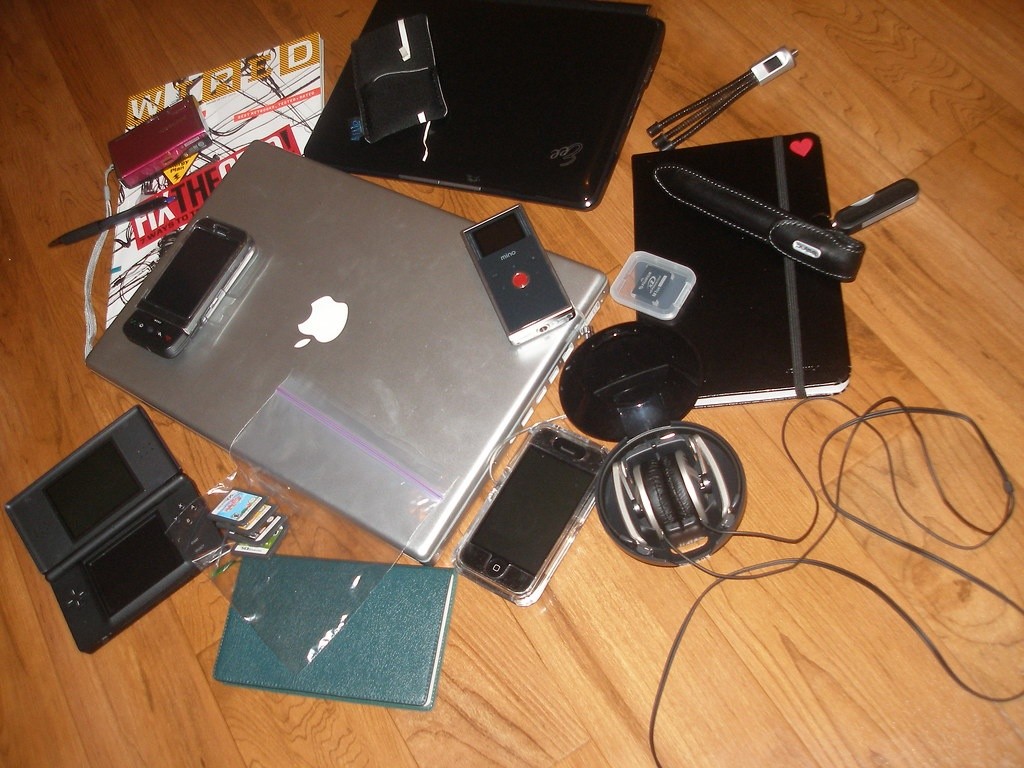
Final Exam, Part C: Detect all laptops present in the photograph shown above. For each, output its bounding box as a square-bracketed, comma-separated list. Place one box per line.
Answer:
[303, 0, 664, 213]
[86, 140, 609, 567]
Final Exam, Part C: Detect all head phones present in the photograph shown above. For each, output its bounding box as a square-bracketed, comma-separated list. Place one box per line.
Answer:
[604, 431, 730, 549]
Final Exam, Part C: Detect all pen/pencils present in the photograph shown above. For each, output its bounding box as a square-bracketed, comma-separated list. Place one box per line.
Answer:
[46, 195, 177, 248]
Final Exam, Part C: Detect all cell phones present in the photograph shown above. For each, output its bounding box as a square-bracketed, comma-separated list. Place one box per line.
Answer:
[454, 422, 609, 606]
[122, 219, 256, 358]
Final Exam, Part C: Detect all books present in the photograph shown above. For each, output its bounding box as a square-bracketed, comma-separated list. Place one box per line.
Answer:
[633, 131, 852, 407]
[104, 32, 325, 331]
[213, 554, 458, 711]
